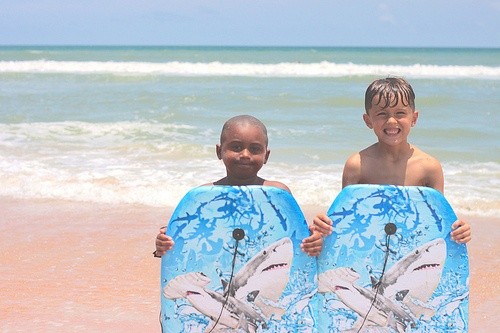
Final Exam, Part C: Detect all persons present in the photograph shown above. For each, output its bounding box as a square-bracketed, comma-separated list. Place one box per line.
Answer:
[314, 78, 472, 244]
[156, 115, 322, 256]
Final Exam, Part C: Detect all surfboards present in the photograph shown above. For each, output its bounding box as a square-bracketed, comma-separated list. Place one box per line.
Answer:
[319, 185, 469, 333]
[160, 186, 318, 333]
